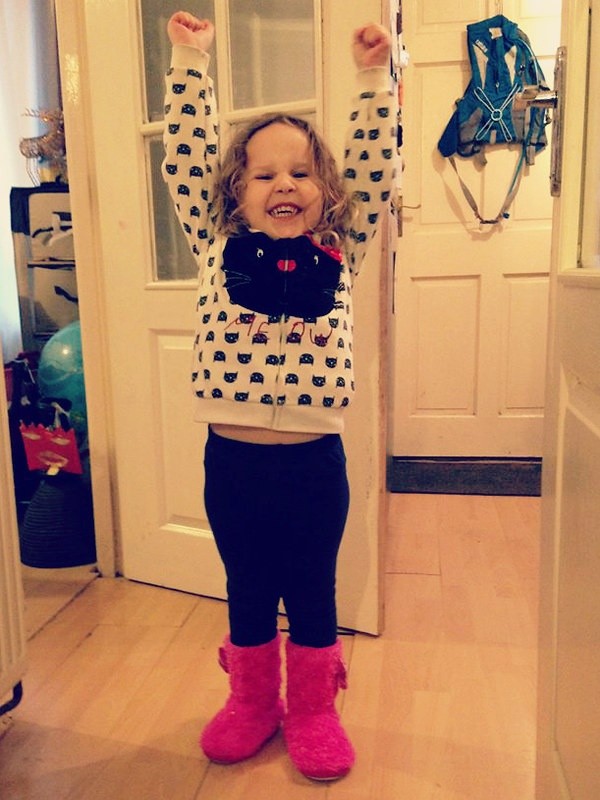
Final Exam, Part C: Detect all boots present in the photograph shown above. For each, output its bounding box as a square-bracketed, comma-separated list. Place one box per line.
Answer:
[281, 636, 356, 780]
[199, 629, 283, 763]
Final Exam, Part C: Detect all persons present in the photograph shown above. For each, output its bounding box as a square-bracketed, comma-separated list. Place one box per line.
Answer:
[161, 10, 399, 781]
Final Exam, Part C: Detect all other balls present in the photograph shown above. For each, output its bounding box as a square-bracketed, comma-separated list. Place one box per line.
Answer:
[37, 320, 88, 433]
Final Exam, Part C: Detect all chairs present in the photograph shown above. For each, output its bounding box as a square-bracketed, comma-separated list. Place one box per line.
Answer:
[27, 193, 77, 238]
[11, 226, 76, 352]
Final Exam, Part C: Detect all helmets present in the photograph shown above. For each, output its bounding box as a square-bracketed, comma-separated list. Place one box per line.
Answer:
[37, 320, 88, 433]
